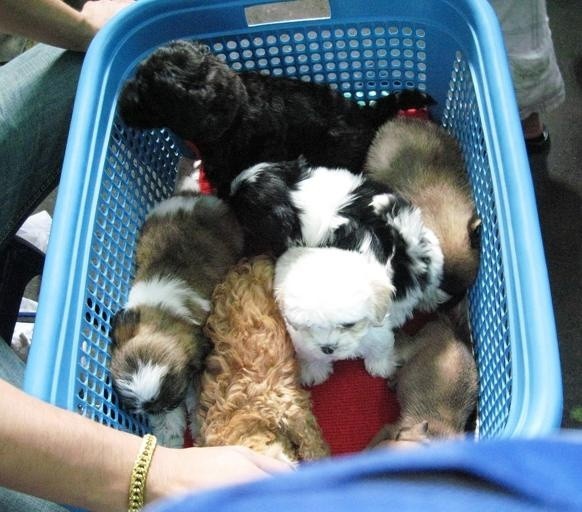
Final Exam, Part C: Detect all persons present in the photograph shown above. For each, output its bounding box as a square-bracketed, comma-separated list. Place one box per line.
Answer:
[486, 0, 567, 154]
[1, 2, 297, 512]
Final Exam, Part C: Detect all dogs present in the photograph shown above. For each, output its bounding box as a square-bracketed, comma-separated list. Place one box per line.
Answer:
[365, 114, 484, 312]
[357, 310, 481, 454]
[114, 39, 439, 198]
[108, 190, 242, 450]
[273, 241, 398, 388]
[197, 253, 332, 464]
[226, 154, 453, 319]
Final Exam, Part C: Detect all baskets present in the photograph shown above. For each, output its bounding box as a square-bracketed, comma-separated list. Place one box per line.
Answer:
[24, 0, 564, 440]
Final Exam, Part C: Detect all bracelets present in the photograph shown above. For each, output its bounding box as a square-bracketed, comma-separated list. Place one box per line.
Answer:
[127, 434, 158, 512]
